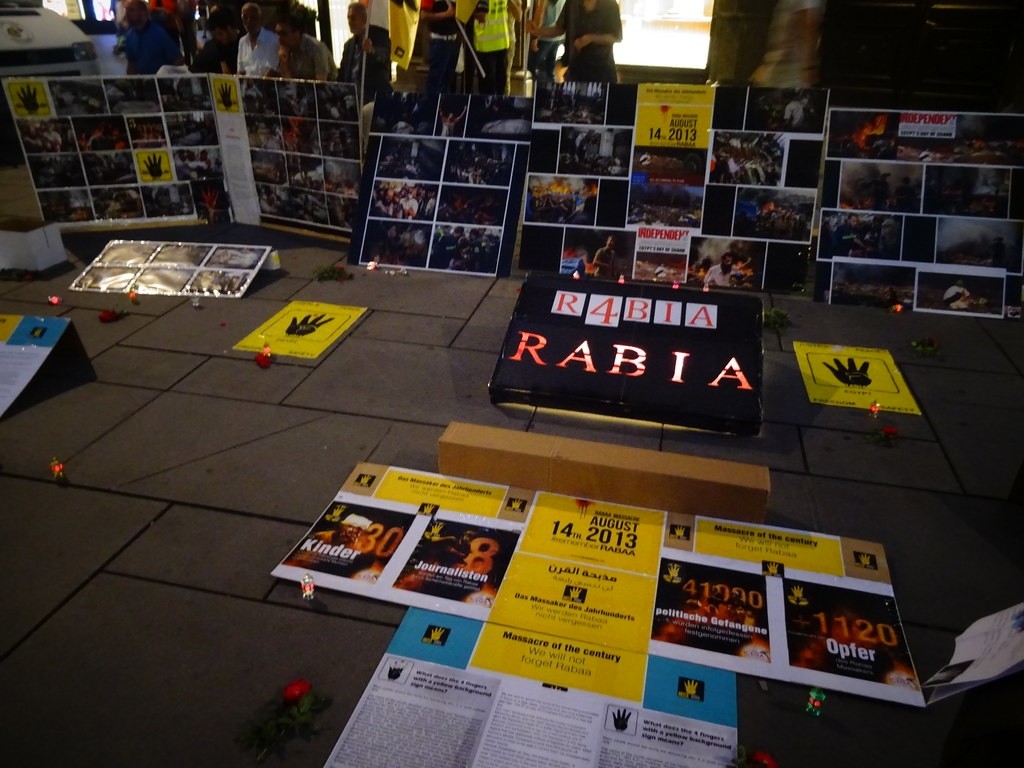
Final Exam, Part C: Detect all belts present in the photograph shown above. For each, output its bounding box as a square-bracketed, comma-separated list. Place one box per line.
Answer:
[430, 32, 457, 42]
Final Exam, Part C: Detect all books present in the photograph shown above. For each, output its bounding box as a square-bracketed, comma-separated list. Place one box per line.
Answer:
[231, 300, 372, 367]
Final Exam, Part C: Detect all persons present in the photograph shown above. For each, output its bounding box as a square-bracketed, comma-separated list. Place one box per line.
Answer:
[471, 0, 523, 96]
[527, 0, 623, 85]
[340, 3, 393, 104]
[113, 0, 245, 76]
[237, 3, 281, 76]
[781, 91, 811, 130]
[591, 235, 618, 282]
[569, 178, 599, 227]
[942, 278, 968, 309]
[712, 130, 778, 183]
[418, 0, 462, 98]
[364, 138, 506, 275]
[818, 211, 900, 262]
[242, 81, 362, 232]
[655, 261, 669, 281]
[265, 16, 339, 82]
[438, 105, 466, 137]
[698, 241, 761, 290]
[736, 185, 804, 238]
[981, 236, 1007, 268]
[24, 115, 224, 224]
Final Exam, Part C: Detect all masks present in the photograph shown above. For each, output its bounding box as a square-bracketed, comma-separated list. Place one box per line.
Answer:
[406, 193, 412, 199]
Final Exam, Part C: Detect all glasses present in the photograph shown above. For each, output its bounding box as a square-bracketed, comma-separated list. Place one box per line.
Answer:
[274, 30, 292, 36]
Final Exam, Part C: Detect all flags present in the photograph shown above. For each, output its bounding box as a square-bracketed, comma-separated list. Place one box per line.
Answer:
[390, 0, 421, 70]
